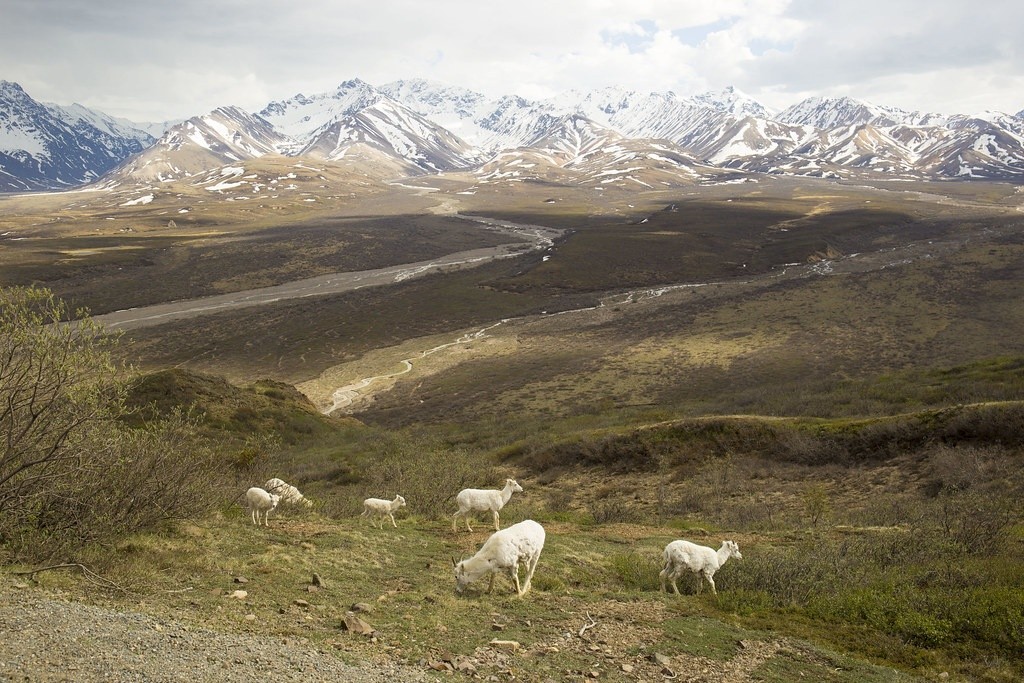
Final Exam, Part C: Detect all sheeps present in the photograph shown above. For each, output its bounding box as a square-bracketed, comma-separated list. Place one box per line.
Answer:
[265, 478, 313, 509]
[361, 494, 407, 529]
[659, 537, 742, 595]
[451, 519, 546, 596]
[247, 487, 283, 527]
[451, 476, 523, 531]
